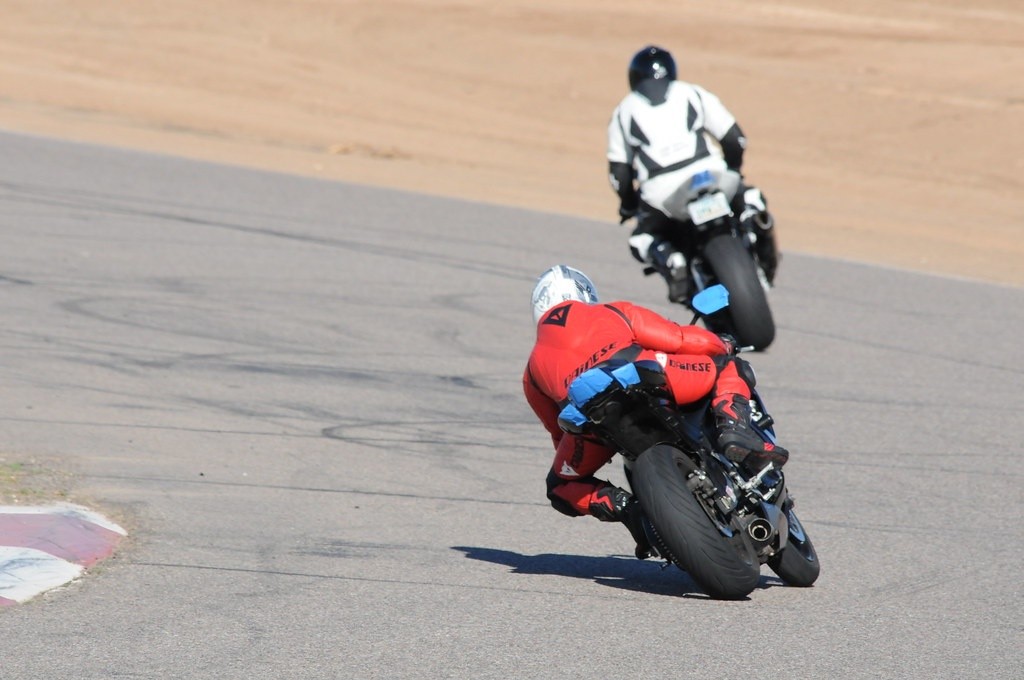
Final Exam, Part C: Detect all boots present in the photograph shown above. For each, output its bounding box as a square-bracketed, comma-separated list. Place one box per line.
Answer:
[711, 394, 788, 473]
[589, 481, 653, 560]
[649, 237, 688, 301]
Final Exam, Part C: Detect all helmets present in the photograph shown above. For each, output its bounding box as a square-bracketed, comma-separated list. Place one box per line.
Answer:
[629, 47, 676, 91]
[531, 265, 598, 326]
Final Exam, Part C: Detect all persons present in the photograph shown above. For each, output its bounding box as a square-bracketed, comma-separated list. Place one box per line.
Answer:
[606, 45, 779, 303]
[520, 264, 789, 522]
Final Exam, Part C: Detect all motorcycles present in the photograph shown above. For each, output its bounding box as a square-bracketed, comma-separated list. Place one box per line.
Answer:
[604, 283, 821, 599]
[619, 164, 778, 351]
